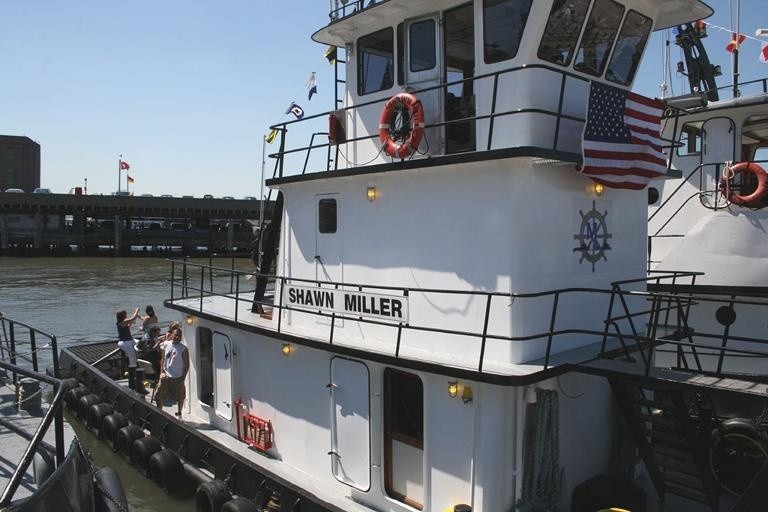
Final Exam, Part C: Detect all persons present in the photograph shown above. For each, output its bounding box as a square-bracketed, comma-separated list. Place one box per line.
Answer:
[154, 326, 190, 416]
[165, 320, 182, 341]
[137, 325, 163, 386]
[115, 306, 141, 388]
[141, 304, 158, 333]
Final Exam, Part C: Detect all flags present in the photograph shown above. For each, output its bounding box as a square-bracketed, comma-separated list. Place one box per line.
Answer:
[726, 31, 746, 54]
[120, 161, 130, 170]
[127, 176, 134, 183]
[264, 128, 280, 143]
[306, 72, 318, 101]
[759, 41, 768, 64]
[693, 20, 708, 39]
[323, 45, 337, 66]
[284, 101, 304, 120]
[573, 77, 669, 191]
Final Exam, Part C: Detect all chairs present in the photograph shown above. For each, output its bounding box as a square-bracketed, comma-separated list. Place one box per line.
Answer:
[136, 358, 160, 403]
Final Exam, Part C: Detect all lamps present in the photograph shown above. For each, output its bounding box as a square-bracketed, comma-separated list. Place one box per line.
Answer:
[186, 314, 193, 325]
[462, 385, 474, 403]
[365, 185, 375, 202]
[446, 381, 458, 400]
[594, 182, 603, 195]
[281, 341, 291, 356]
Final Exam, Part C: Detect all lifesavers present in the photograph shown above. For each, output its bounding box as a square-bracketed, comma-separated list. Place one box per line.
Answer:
[379, 93, 424, 158]
[722, 163, 768, 206]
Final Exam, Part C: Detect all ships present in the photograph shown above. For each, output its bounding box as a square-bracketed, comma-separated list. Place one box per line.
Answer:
[44, 2, 767, 510]
[644, 1, 768, 382]
